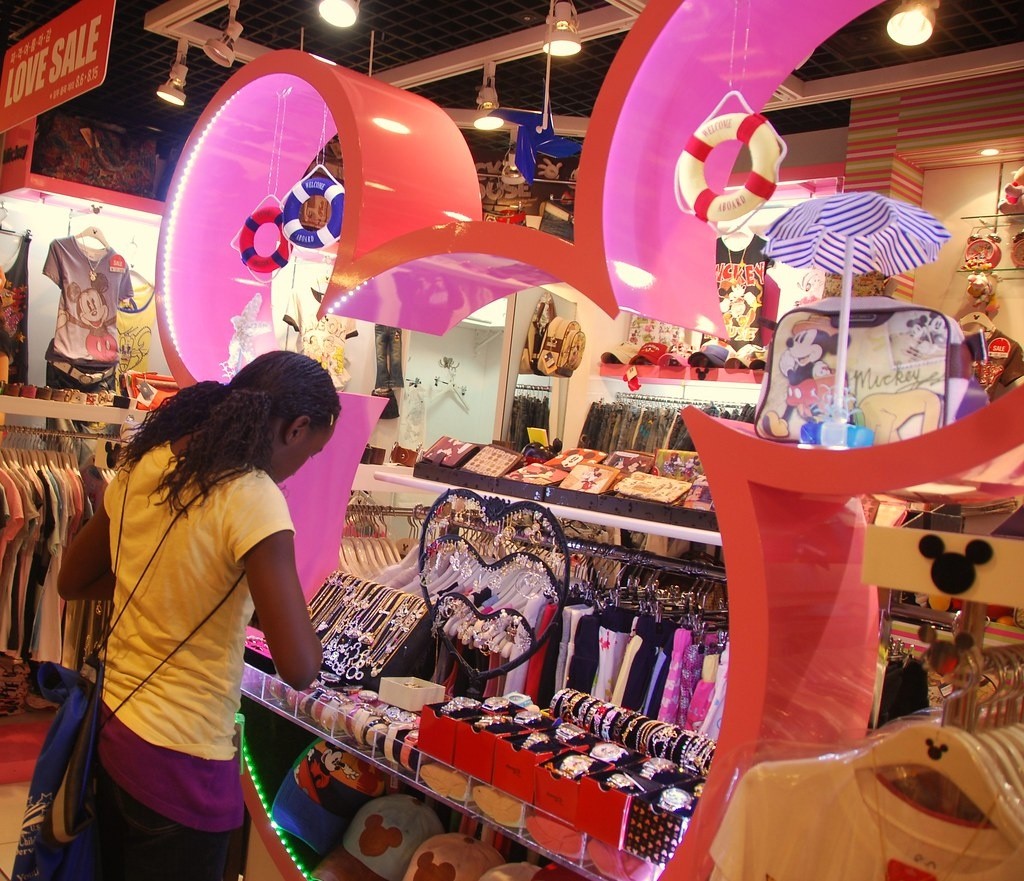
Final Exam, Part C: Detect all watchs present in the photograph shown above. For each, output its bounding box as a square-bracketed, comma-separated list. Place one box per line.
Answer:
[440, 697, 694, 812]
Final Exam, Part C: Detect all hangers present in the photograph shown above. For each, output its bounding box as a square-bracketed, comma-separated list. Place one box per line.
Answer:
[596, 391, 758, 421]
[337, 504, 729, 658]
[510, 383, 554, 409]
[0, 207, 33, 241]
[431, 366, 469, 412]
[72, 227, 111, 253]
[956, 303, 995, 334]
[853, 611, 1024, 848]
[0, 425, 118, 481]
[722, 222, 754, 240]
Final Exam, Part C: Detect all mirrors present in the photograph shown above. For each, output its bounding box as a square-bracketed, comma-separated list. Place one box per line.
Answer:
[505, 292, 556, 449]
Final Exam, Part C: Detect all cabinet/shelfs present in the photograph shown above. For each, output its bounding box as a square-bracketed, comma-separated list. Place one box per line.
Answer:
[955, 214, 1024, 281]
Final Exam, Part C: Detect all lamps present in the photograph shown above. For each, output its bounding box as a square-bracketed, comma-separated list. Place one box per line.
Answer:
[543, 0, 582, 56]
[319, 0, 360, 28]
[501, 126, 526, 185]
[157, 39, 189, 106]
[474, 61, 504, 131]
[202, 0, 243, 67]
[887, 0, 939, 46]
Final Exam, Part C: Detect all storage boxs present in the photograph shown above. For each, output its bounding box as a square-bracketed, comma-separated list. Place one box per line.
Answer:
[377, 677, 705, 867]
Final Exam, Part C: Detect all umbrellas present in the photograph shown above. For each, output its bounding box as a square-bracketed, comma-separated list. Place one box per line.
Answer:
[763, 190, 952, 409]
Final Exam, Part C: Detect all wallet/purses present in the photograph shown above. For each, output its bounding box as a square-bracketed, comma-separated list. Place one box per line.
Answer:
[422, 431, 716, 514]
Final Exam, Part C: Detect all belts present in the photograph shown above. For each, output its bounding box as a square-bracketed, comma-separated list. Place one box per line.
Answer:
[47, 356, 116, 387]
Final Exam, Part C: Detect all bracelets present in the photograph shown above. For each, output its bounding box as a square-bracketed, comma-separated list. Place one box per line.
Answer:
[270, 672, 419, 774]
[549, 687, 717, 778]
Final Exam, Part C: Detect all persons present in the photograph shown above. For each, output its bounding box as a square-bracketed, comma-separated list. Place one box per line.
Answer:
[57, 350, 342, 881]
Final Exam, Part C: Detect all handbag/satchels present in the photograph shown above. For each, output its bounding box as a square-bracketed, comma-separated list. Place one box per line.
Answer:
[750, 298, 985, 450]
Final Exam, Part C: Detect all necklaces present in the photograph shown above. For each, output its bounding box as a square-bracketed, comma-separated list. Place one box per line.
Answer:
[81, 232, 109, 281]
[306, 570, 428, 681]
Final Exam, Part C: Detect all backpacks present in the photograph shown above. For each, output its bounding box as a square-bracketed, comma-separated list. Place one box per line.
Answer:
[6, 647, 99, 881]
[529, 298, 585, 379]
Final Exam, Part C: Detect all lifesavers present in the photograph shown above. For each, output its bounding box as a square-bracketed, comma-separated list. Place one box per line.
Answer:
[238, 205, 294, 275]
[677, 112, 782, 223]
[280, 177, 345, 250]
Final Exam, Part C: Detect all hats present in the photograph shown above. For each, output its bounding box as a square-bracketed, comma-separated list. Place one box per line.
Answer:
[403, 832, 586, 881]
[311, 792, 445, 881]
[270, 738, 387, 854]
[601, 338, 768, 372]
